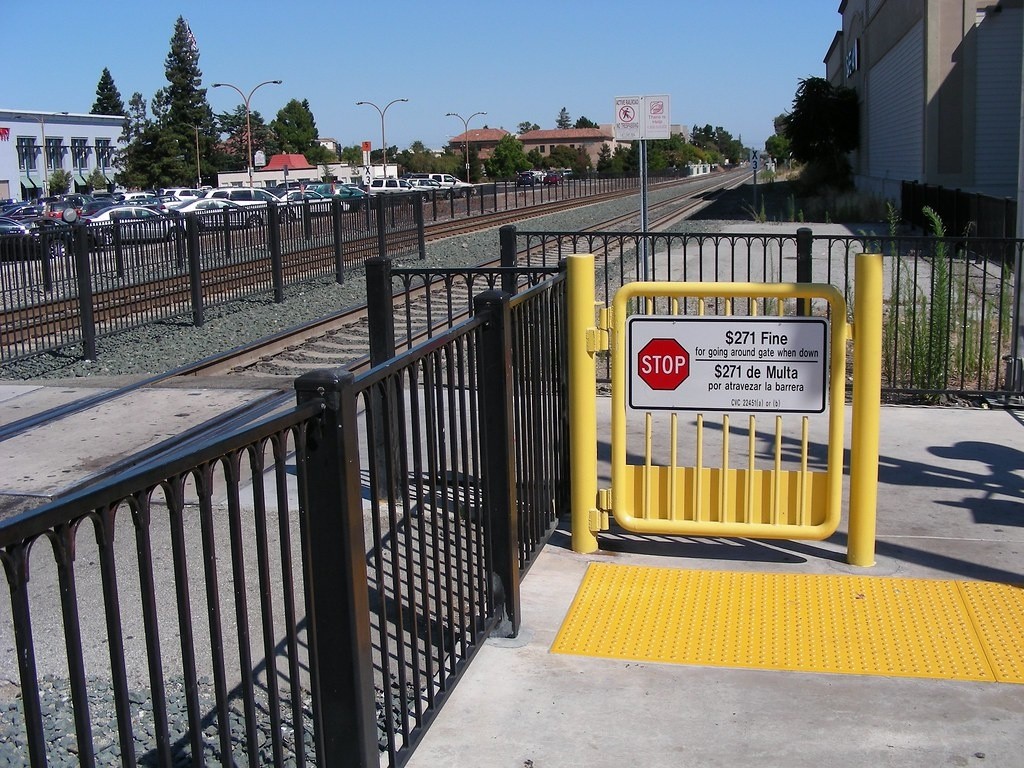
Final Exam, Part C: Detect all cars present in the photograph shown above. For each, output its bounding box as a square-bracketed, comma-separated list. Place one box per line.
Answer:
[516, 168, 573, 187]
[0, 173, 477, 261]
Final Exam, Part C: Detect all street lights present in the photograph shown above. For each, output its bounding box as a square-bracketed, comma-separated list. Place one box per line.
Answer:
[178, 122, 213, 188]
[355, 98, 408, 179]
[445, 111, 487, 182]
[15, 111, 69, 197]
[211, 80, 282, 187]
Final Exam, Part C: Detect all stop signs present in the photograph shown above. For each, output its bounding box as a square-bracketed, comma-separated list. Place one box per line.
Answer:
[637, 338, 690, 391]
